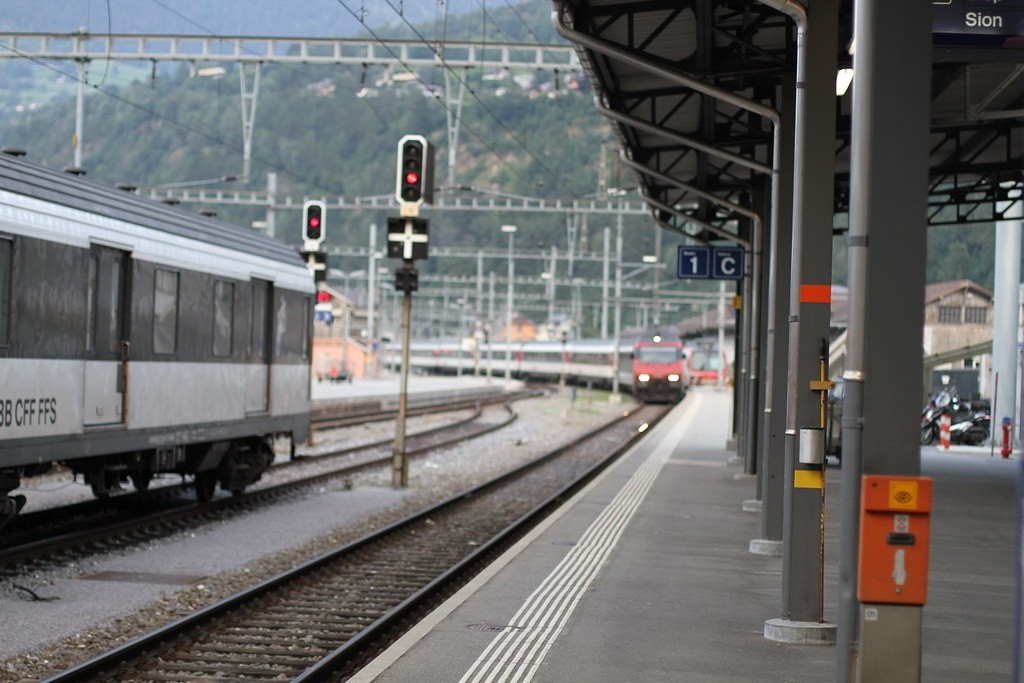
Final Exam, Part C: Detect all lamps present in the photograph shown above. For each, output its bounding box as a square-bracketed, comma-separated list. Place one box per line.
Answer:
[836, 67, 855, 97]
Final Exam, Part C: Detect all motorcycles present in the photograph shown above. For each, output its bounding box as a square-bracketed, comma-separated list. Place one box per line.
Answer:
[920, 386, 991, 447]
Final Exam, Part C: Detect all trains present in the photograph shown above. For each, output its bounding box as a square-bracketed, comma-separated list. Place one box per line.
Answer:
[681, 342, 729, 386]
[0, 143, 315, 551]
[377, 330, 689, 404]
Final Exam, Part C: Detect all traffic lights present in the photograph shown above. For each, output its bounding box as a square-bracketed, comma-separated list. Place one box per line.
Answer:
[302, 199, 325, 243]
[395, 135, 427, 206]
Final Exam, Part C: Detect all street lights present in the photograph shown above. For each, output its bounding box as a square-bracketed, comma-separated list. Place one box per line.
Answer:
[500, 224, 518, 382]
